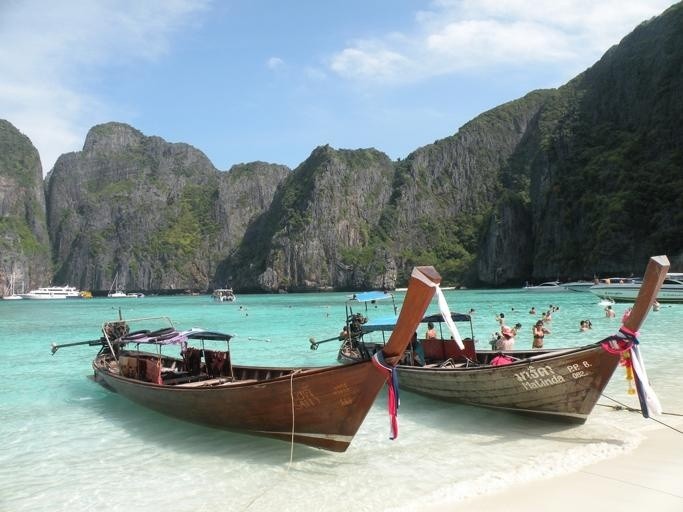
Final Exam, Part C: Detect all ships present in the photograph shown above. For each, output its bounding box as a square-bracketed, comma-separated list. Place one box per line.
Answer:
[17, 284, 78, 299]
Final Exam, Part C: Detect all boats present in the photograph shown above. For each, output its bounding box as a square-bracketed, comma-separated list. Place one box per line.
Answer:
[522, 280, 567, 293]
[558, 276, 642, 292]
[79, 290, 93, 298]
[210, 287, 235, 304]
[64, 294, 84, 300]
[588, 273, 682, 304]
[127, 292, 144, 297]
[48, 263, 441, 456]
[310, 254, 671, 426]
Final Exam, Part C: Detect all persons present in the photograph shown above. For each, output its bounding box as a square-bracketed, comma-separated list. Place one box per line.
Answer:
[511, 323, 522, 335]
[532, 319, 551, 348]
[496, 313, 504, 324]
[496, 325, 514, 350]
[426, 322, 436, 340]
[653, 300, 660, 311]
[593, 273, 624, 285]
[409, 332, 425, 366]
[580, 319, 592, 330]
[604, 305, 615, 318]
[529, 304, 560, 321]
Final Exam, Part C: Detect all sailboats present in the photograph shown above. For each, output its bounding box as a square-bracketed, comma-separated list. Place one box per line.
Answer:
[105, 271, 126, 297]
[3, 275, 21, 299]
